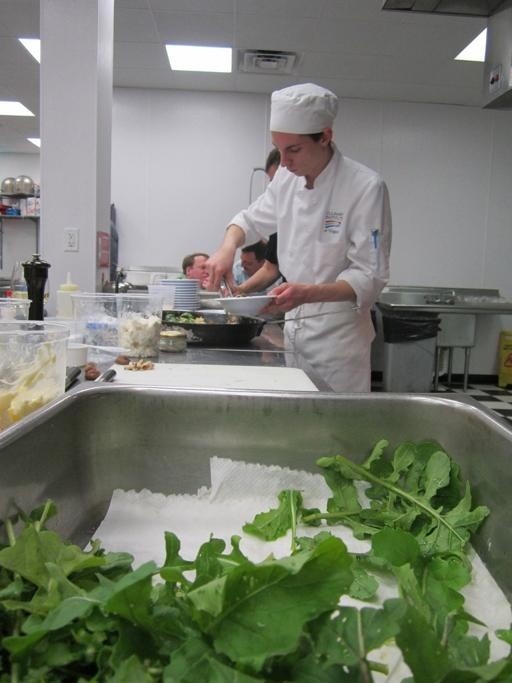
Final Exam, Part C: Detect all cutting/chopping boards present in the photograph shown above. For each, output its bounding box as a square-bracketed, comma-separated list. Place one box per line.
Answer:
[95, 363, 318, 393]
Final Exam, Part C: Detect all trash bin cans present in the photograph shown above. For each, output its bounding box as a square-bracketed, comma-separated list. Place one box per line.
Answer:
[382, 309, 442, 393]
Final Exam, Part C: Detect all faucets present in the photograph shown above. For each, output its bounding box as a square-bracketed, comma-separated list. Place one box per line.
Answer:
[440, 288, 459, 304]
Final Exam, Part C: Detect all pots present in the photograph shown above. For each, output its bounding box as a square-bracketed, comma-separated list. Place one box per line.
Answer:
[158, 308, 365, 347]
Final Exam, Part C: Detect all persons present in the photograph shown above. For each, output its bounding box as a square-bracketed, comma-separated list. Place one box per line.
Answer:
[223, 239, 284, 297]
[202, 80, 393, 393]
[222, 148, 289, 298]
[166, 251, 212, 292]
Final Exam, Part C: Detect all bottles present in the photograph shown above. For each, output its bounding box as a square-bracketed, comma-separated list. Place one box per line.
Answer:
[56, 273, 81, 319]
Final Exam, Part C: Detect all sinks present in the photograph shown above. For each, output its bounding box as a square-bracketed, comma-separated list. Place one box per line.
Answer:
[376, 290, 460, 308]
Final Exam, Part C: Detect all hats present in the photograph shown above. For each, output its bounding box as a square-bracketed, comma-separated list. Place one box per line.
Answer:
[268, 80, 340, 137]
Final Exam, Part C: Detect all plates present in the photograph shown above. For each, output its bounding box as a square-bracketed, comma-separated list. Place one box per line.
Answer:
[156, 279, 198, 310]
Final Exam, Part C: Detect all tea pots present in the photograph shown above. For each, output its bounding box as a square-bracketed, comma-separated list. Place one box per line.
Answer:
[104, 271, 127, 294]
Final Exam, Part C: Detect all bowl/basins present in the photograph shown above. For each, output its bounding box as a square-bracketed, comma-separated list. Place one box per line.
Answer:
[0, 176, 35, 195]
[199, 295, 281, 321]
